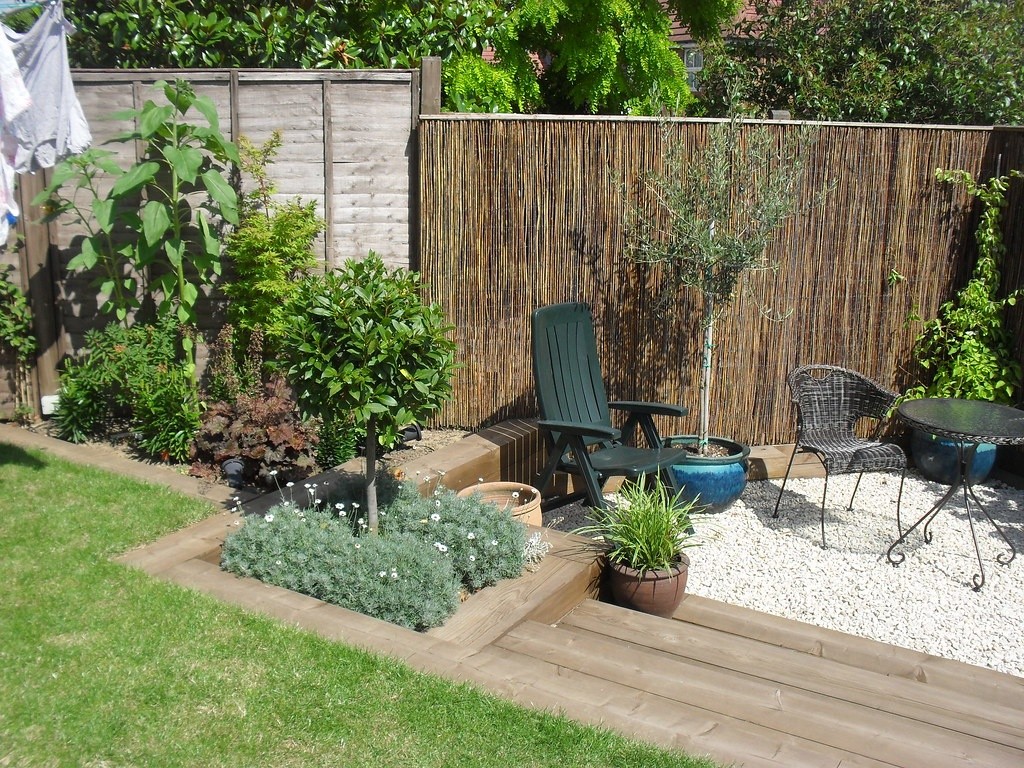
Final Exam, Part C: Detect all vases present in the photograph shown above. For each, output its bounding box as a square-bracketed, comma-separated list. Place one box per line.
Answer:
[452, 482, 545, 529]
[221, 460, 245, 490]
[401, 421, 424, 441]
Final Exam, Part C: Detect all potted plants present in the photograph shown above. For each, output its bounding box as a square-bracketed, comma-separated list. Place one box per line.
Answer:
[886, 155, 1024, 489]
[603, 76, 840, 514]
[567, 467, 718, 618]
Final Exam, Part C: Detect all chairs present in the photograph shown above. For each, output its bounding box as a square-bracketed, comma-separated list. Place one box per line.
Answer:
[528, 301, 698, 542]
[770, 364, 909, 551]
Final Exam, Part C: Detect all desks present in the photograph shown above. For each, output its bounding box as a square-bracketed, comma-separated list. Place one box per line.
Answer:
[887, 399, 1024, 589]
